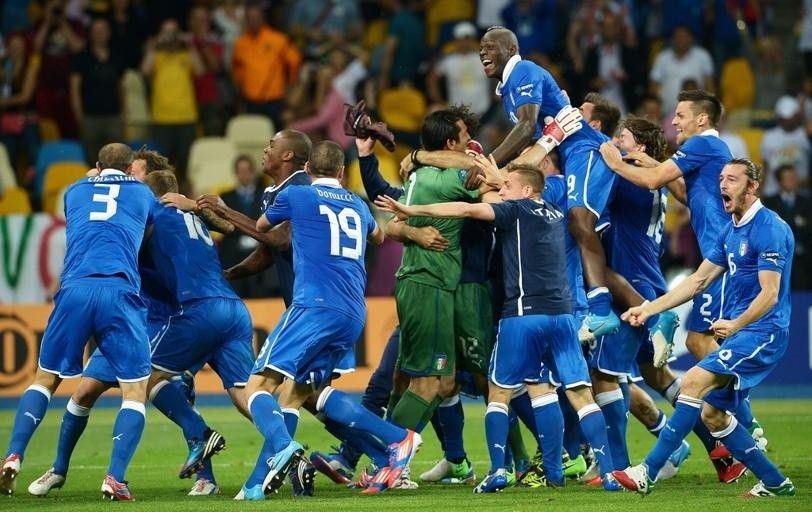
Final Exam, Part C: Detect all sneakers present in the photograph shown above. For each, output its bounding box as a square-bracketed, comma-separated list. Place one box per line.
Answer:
[27, 468, 66, 496]
[419, 456, 477, 485]
[178, 429, 225, 480]
[261, 441, 305, 496]
[575, 309, 622, 342]
[710, 416, 763, 459]
[311, 441, 354, 484]
[473, 437, 691, 496]
[233, 484, 266, 502]
[0, 454, 21, 495]
[357, 428, 423, 495]
[646, 309, 682, 369]
[288, 455, 318, 497]
[731, 480, 796, 497]
[187, 477, 224, 496]
[101, 474, 137, 503]
[717, 437, 768, 483]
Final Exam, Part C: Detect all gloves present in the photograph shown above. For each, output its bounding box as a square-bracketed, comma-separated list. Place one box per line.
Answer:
[536, 105, 584, 154]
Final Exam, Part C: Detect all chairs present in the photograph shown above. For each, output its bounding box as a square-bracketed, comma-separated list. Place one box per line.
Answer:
[1, 111, 275, 216]
